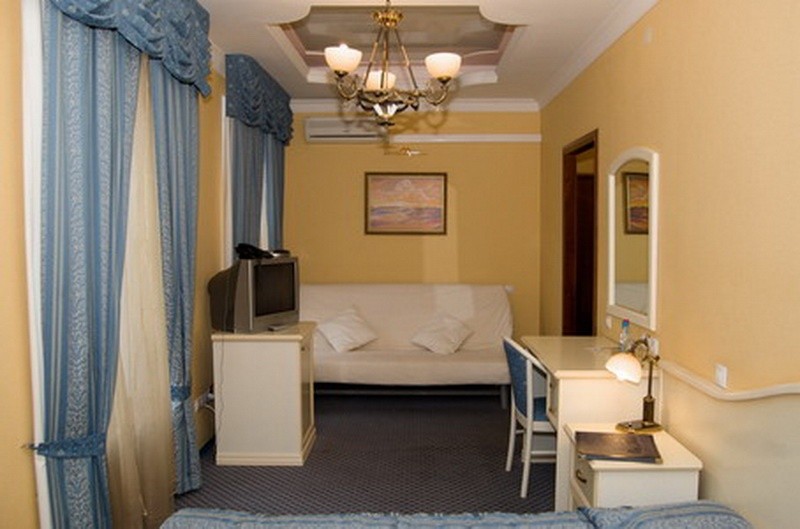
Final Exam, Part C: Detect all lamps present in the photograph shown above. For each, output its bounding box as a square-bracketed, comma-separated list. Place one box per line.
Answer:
[604, 338, 661, 431]
[324, 0, 461, 127]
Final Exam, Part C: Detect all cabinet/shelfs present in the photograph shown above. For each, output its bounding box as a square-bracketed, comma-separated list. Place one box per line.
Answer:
[211, 320, 318, 466]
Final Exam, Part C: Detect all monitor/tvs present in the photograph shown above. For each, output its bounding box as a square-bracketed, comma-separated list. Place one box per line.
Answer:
[208, 254, 299, 334]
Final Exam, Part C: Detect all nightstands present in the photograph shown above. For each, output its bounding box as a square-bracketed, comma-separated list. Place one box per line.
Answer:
[562, 422, 705, 509]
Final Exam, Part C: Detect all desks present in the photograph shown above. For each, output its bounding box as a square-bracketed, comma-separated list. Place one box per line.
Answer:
[520, 335, 661, 513]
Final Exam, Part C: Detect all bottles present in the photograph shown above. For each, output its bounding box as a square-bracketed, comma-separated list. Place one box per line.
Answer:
[617, 318, 632, 354]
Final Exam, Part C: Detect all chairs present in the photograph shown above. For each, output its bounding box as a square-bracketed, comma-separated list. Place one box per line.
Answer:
[501, 333, 557, 497]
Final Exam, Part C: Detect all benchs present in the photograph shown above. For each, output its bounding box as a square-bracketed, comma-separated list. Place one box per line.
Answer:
[612, 280, 650, 314]
[299, 283, 515, 408]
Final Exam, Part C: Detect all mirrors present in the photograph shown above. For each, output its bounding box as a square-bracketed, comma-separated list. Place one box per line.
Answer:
[607, 142, 660, 331]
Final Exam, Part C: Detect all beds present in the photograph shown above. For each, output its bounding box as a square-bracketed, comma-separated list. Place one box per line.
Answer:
[155, 362, 799, 529]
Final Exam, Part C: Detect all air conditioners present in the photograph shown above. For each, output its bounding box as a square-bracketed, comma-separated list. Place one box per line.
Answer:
[303, 113, 389, 142]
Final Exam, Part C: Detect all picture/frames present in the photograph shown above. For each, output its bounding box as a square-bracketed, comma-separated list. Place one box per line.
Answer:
[364, 171, 448, 235]
[622, 170, 651, 236]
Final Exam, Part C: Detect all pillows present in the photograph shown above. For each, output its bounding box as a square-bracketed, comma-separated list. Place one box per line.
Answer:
[316, 302, 379, 352]
[411, 310, 475, 356]
[578, 500, 755, 528]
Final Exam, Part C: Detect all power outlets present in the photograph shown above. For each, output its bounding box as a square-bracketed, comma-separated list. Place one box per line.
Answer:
[195, 386, 213, 412]
[648, 338, 659, 353]
